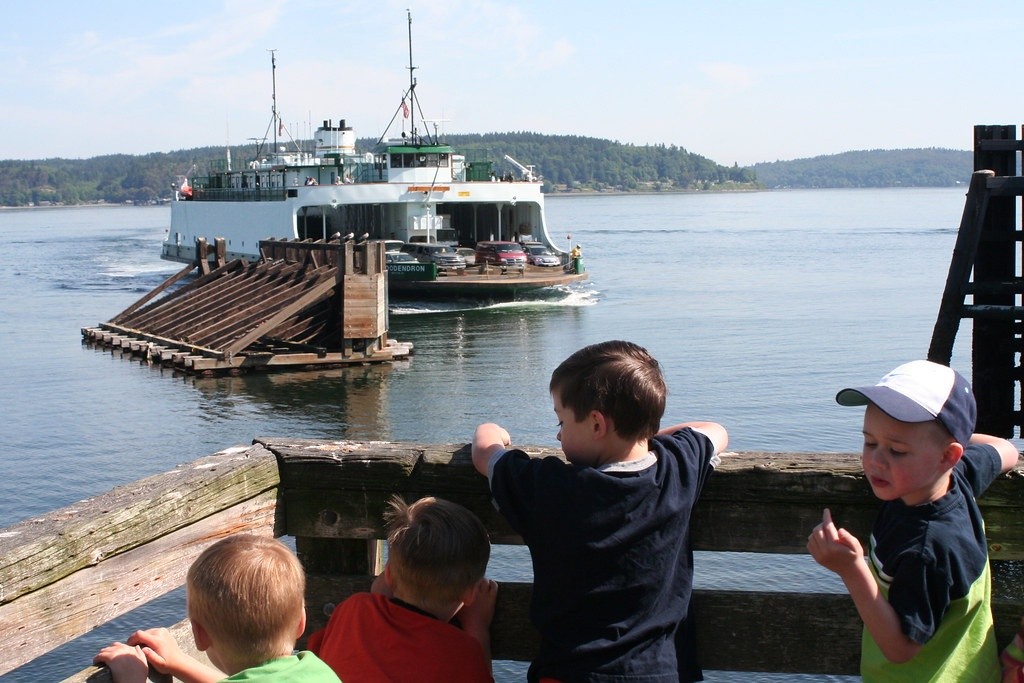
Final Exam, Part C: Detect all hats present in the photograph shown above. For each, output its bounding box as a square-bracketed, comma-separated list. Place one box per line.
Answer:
[835, 359, 976, 449]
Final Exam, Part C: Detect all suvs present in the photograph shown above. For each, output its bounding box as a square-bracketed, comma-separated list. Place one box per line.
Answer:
[400, 243, 467, 273]
[374, 238, 404, 254]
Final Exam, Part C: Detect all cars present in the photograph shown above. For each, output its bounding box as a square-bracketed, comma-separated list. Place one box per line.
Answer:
[520, 245, 561, 267]
[385, 251, 420, 264]
[454, 248, 477, 266]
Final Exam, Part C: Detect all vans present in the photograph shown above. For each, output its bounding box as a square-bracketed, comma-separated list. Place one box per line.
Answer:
[475, 241, 527, 267]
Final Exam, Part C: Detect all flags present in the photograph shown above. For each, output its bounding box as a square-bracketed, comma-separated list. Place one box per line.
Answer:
[403, 102, 410, 118]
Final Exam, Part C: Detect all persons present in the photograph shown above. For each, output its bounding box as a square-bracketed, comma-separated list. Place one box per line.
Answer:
[471, 340, 728, 683]
[572, 244, 581, 255]
[490, 171, 514, 182]
[95, 533, 343, 683]
[305, 176, 316, 186]
[320, 497, 500, 683]
[335, 175, 355, 185]
[808, 360, 1019, 682]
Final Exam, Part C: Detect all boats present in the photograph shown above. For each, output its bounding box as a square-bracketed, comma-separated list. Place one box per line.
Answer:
[181, 176, 203, 198]
[161, 6, 588, 303]
